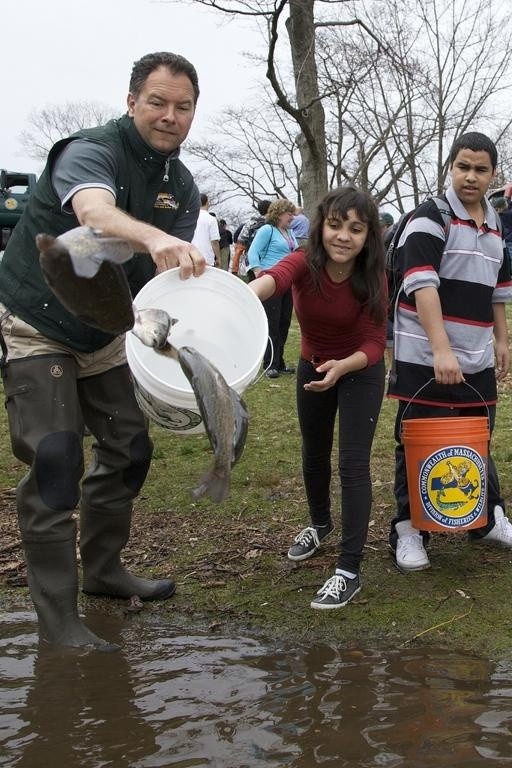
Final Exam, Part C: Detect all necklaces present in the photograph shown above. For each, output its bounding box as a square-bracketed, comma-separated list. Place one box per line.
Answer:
[329, 262, 352, 276]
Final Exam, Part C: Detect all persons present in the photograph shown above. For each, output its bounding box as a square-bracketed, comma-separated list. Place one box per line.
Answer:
[246, 187, 389, 610]
[386, 132, 512, 572]
[1, 52, 205, 653]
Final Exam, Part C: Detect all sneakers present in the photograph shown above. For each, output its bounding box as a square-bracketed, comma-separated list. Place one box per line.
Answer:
[264, 366, 296, 379]
[482, 503, 512, 547]
[389, 517, 431, 573]
[311, 571, 362, 612]
[287, 522, 335, 561]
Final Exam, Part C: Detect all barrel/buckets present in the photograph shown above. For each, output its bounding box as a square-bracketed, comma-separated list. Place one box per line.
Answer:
[399, 377, 492, 533]
[125, 266, 275, 436]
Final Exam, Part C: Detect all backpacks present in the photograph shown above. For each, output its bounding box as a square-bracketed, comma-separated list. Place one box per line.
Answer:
[384, 198, 452, 322]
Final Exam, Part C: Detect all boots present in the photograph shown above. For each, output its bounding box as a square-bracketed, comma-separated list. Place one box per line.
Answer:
[22, 527, 122, 655]
[77, 498, 179, 602]
